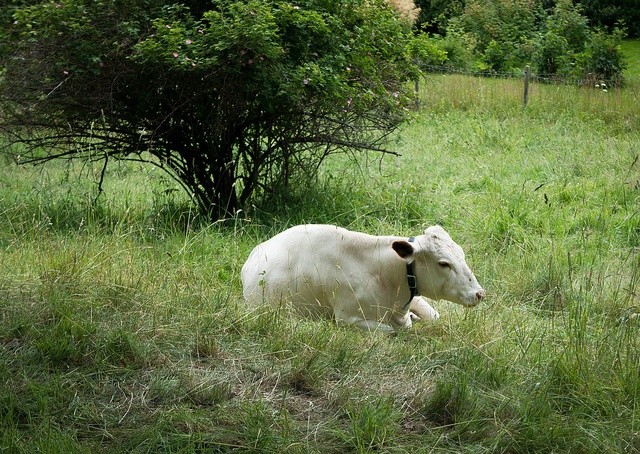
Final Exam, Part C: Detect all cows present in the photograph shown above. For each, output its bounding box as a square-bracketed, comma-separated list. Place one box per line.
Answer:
[241, 224, 485, 333]
